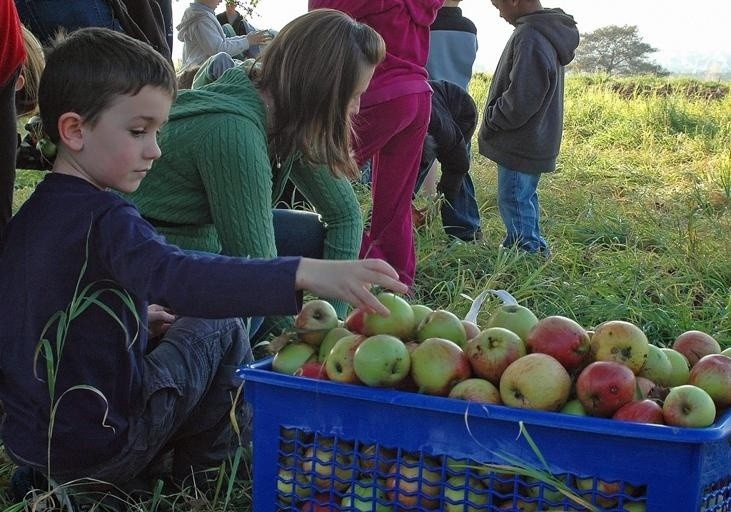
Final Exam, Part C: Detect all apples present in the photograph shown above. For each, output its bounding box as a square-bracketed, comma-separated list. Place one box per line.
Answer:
[37, 137, 56, 158]
[270, 292, 731, 512]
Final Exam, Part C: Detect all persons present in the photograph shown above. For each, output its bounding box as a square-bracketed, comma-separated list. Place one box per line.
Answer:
[479, 1, 580, 264]
[0, 27, 408, 512]
[1, 0, 483, 348]
